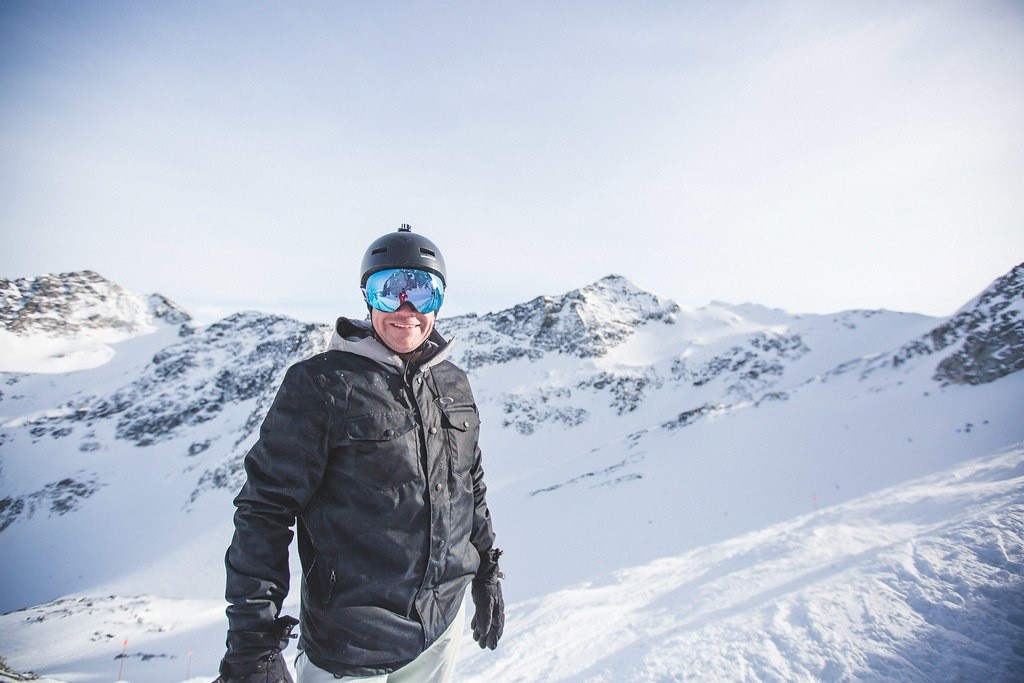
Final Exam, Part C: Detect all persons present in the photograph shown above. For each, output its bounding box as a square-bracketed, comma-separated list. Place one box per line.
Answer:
[399, 288, 408, 304]
[217, 224, 506, 683]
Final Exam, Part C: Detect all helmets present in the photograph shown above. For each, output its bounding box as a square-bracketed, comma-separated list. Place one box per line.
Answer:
[359, 223, 448, 289]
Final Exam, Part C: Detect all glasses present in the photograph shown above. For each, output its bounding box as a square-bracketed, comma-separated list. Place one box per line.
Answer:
[365, 268, 444, 314]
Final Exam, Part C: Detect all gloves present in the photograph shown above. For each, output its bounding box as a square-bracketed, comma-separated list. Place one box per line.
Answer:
[470, 547, 508, 651]
[211, 615, 300, 683]
